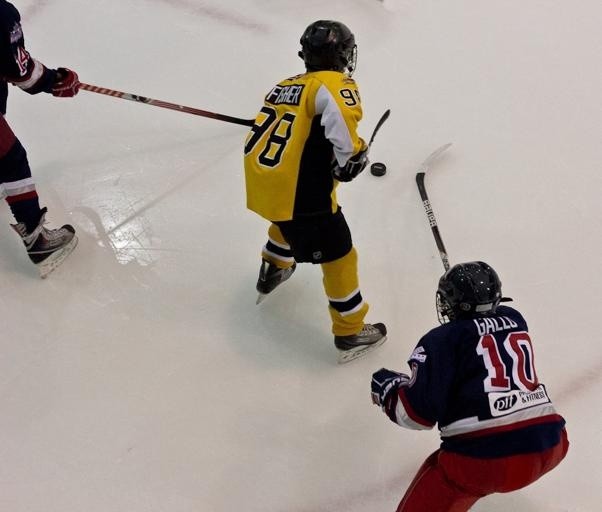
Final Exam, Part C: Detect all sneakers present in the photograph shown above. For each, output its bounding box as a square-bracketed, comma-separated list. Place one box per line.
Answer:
[8, 206, 81, 267]
[255, 250, 299, 295]
[333, 316, 387, 350]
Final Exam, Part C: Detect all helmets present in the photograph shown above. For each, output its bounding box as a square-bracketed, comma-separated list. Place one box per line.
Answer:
[295, 18, 357, 69]
[435, 258, 503, 320]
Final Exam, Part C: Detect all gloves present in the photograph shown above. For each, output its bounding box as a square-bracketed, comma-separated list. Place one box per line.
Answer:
[48, 66, 81, 99]
[368, 367, 410, 406]
[332, 151, 371, 182]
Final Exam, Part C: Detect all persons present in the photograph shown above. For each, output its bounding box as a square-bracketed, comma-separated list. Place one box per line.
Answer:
[2, 2, 80, 268]
[243, 20, 387, 352]
[369, 261, 569, 510]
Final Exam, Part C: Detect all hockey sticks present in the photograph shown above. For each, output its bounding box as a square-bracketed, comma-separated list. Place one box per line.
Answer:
[416, 141, 456, 271]
[368, 110, 390, 145]
